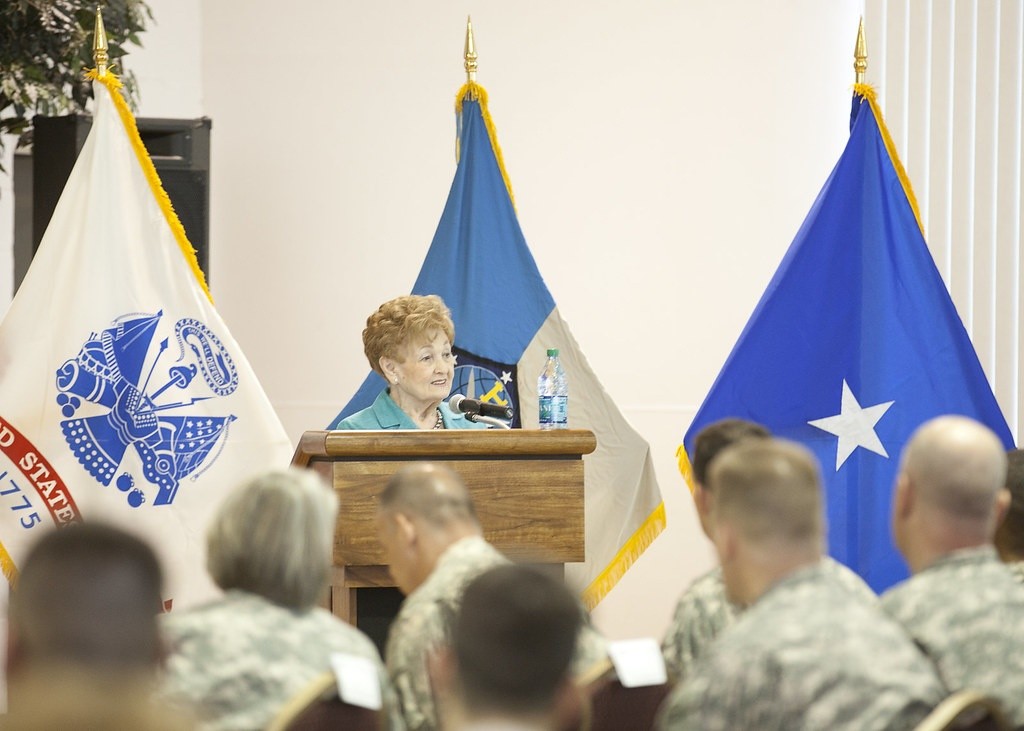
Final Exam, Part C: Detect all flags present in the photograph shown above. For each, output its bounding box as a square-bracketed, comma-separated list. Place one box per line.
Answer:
[323, 82, 666, 617]
[0, 71, 293, 718]
[672, 85, 1017, 594]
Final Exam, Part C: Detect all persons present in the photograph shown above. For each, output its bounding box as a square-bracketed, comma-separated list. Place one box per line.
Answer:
[878, 413, 1024, 731]
[432, 565, 585, 730]
[146, 463, 409, 730]
[0, 519, 196, 730]
[994, 449, 1024, 588]
[661, 418, 772, 688]
[336, 293, 487, 430]
[647, 435, 949, 730]
[376, 461, 616, 730]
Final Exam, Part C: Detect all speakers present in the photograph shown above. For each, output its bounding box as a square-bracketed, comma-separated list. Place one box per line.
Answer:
[31, 112, 212, 293]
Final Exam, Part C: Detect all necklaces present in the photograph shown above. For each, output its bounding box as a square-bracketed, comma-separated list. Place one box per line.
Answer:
[430, 409, 443, 429]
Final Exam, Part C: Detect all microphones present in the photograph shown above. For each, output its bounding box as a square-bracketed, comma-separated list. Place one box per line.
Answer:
[448, 394, 515, 420]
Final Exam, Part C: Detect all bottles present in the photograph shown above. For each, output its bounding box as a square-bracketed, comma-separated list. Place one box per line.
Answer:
[537, 349, 569, 429]
